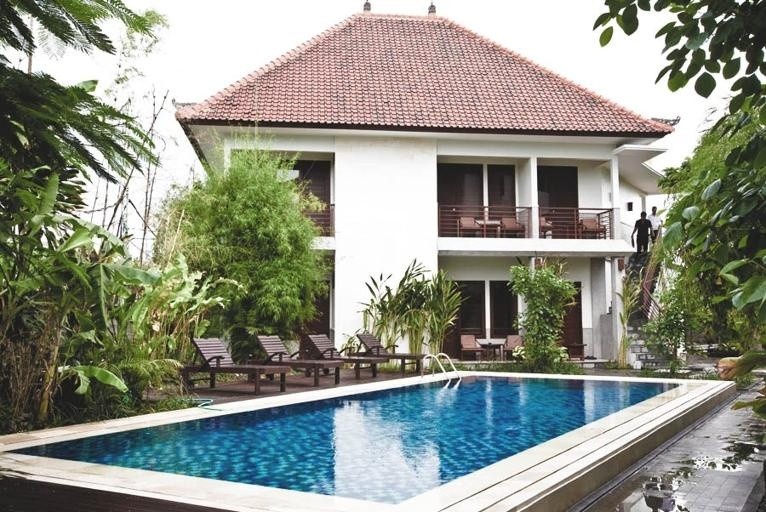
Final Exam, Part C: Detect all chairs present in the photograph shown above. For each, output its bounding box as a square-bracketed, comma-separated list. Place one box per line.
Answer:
[458, 216, 526, 238]
[540, 214, 608, 238]
[181, 329, 425, 392]
[459, 332, 523, 362]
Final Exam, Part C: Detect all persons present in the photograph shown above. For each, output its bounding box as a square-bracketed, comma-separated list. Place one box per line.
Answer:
[631, 211, 654, 252]
[647, 206, 663, 246]
